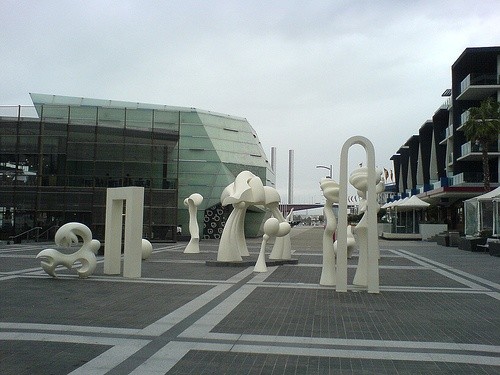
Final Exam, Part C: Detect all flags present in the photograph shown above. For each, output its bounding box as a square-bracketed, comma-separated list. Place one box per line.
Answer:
[391, 170, 393, 180]
[384, 168, 389, 179]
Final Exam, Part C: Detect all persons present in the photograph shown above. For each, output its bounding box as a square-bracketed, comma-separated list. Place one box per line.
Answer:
[177, 224, 182, 242]
